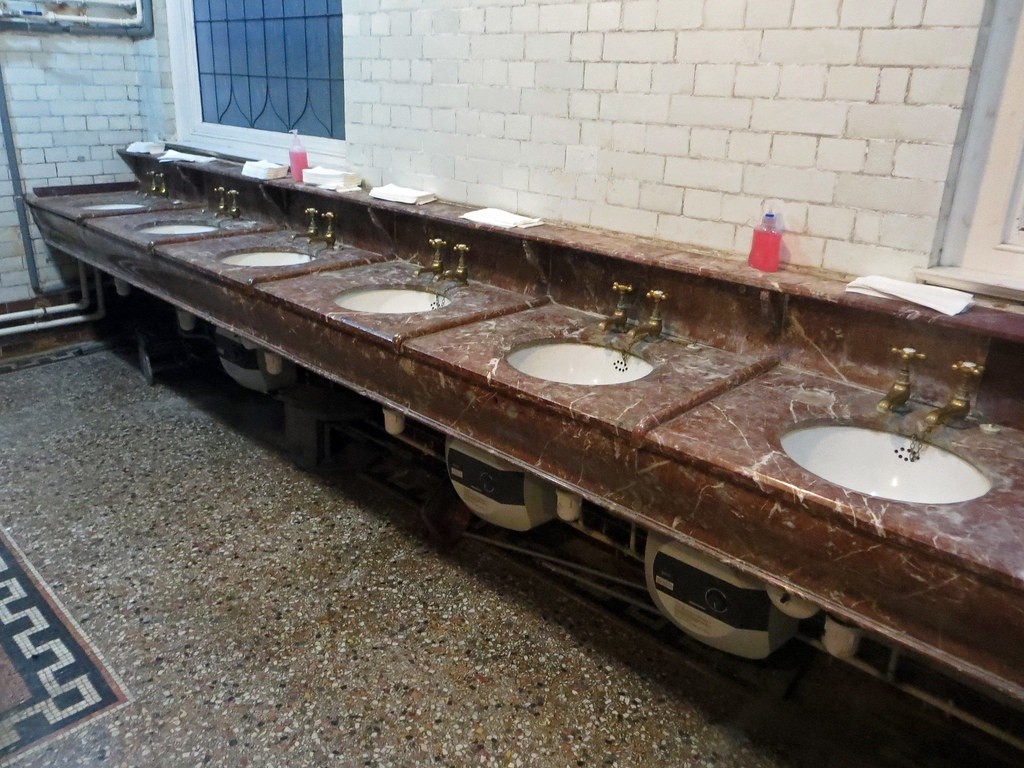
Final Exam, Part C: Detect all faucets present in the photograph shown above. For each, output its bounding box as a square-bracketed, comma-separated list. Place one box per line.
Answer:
[202, 186, 227, 214]
[915, 354, 988, 442]
[289, 207, 320, 241]
[412, 237, 447, 279]
[623, 290, 670, 354]
[874, 340, 924, 415]
[307, 212, 334, 249]
[145, 173, 169, 199]
[136, 170, 158, 192]
[427, 244, 469, 286]
[598, 283, 636, 331]
[215, 190, 240, 220]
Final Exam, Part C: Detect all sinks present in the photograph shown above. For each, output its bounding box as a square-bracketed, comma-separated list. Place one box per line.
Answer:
[135, 221, 219, 234]
[768, 417, 993, 505]
[216, 247, 318, 267]
[333, 285, 453, 314]
[502, 335, 655, 385]
[82, 203, 146, 210]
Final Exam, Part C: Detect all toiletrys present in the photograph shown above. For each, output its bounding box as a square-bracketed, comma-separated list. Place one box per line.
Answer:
[287, 129, 308, 181]
[748, 208, 782, 272]
